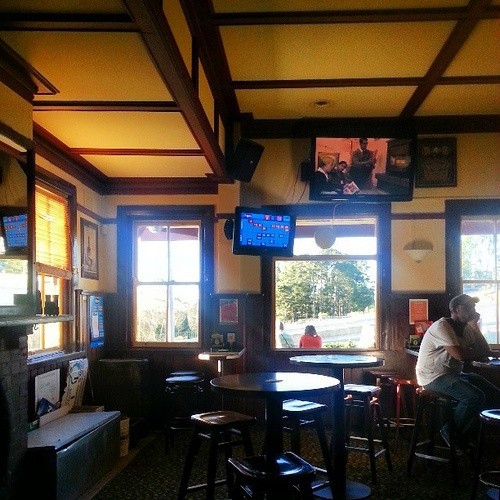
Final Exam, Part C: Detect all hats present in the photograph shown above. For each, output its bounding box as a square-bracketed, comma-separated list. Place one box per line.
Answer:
[449, 294, 480, 310]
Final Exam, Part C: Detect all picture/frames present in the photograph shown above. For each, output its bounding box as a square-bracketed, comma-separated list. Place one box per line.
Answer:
[413, 136, 458, 191]
[79, 217, 101, 282]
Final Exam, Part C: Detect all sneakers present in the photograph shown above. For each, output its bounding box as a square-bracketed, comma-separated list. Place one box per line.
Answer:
[439, 425, 466, 457]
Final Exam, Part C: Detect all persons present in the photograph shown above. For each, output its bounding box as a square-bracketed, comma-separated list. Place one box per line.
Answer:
[314, 154, 348, 194]
[414, 293, 500, 455]
[280, 322, 295, 348]
[351, 137, 377, 188]
[299, 325, 322, 347]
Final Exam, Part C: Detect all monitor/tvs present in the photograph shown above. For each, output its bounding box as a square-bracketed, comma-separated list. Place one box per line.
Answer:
[0, 207, 29, 256]
[308, 131, 416, 204]
[231, 206, 297, 258]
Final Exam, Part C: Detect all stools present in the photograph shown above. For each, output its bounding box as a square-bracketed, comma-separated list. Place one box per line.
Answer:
[161, 366, 203, 437]
[404, 386, 461, 489]
[282, 398, 333, 500]
[369, 371, 404, 439]
[176, 409, 257, 500]
[224, 451, 317, 500]
[344, 384, 396, 488]
[464, 407, 500, 500]
[385, 377, 425, 443]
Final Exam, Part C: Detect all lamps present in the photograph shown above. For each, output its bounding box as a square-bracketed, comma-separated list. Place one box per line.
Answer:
[403, 247, 432, 266]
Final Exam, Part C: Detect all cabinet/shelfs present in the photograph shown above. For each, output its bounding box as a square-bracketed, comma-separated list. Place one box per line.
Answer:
[0, 39, 42, 316]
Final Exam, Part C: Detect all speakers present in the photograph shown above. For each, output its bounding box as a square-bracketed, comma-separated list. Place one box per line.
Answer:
[227, 138, 265, 183]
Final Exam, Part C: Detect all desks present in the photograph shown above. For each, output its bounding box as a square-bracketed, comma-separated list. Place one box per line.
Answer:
[207, 371, 343, 500]
[401, 344, 500, 453]
[288, 353, 384, 456]
[198, 345, 249, 410]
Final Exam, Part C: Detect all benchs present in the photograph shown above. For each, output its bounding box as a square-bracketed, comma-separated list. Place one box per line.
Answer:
[27, 411, 120, 500]
